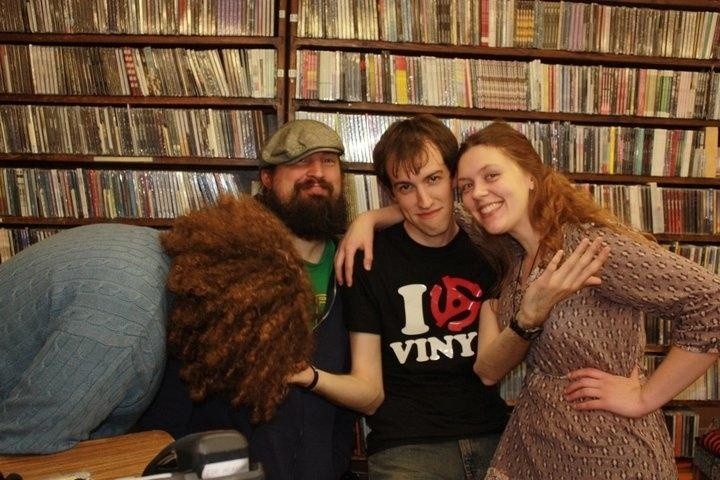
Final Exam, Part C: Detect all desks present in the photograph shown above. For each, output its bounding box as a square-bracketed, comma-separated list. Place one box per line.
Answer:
[0, 431, 179, 479]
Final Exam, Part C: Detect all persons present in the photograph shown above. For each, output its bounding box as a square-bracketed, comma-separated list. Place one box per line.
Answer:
[283, 116, 613, 478]
[252, 119, 354, 479]
[0, 193, 318, 456]
[333, 122, 719, 479]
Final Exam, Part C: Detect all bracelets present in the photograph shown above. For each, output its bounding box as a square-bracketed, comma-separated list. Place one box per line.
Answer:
[304, 363, 319, 391]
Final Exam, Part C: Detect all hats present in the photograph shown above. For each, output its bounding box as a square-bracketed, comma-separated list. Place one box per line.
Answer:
[258, 119, 345, 167]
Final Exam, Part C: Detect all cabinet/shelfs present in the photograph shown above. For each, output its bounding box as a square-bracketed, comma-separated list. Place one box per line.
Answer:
[287, 2, 718, 273]
[0, 0, 287, 264]
[642, 310, 719, 479]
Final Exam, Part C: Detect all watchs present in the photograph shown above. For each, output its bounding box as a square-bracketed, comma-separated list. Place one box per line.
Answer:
[507, 310, 544, 341]
